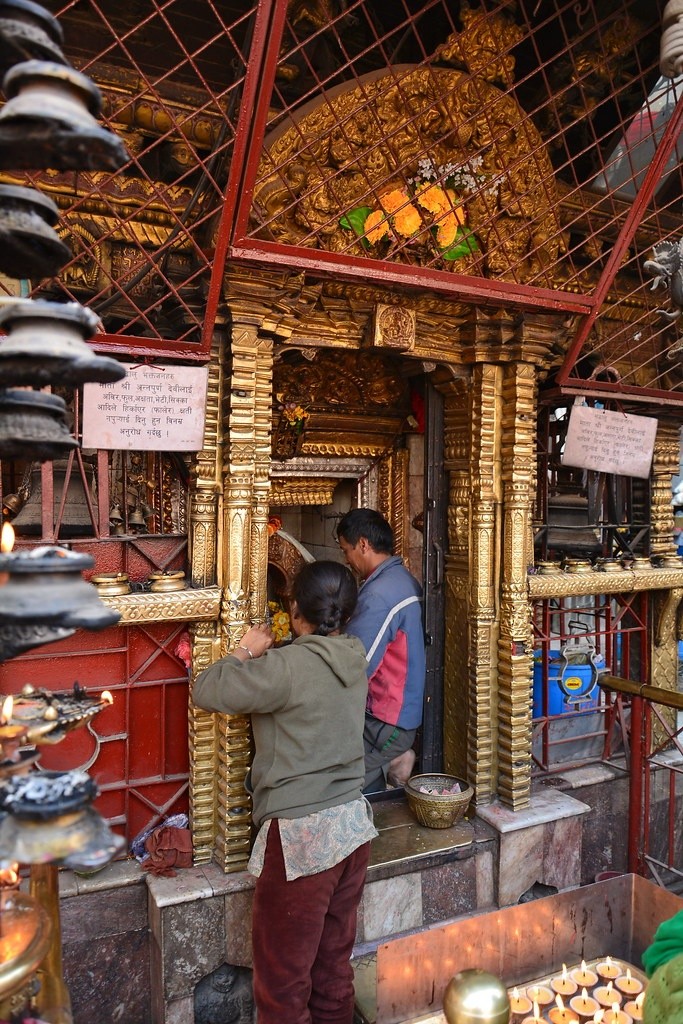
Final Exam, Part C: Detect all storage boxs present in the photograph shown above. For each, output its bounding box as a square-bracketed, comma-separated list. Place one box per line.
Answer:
[375, 873, 683, 1024]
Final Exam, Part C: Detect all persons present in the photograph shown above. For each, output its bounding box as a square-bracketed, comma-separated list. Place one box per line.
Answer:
[190, 560, 380, 1024]
[336, 507, 427, 794]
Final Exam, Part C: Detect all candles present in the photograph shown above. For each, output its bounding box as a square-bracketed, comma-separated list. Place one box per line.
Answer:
[570, 986, 601, 1024]
[521, 997, 549, 1024]
[570, 960, 599, 991]
[585, 1009, 606, 1024]
[593, 981, 623, 1011]
[603, 1002, 633, 1024]
[614, 968, 643, 1001]
[623, 992, 646, 1024]
[526, 984, 555, 1010]
[549, 963, 578, 1003]
[547, 993, 579, 1024]
[508, 987, 532, 1019]
[596, 956, 623, 986]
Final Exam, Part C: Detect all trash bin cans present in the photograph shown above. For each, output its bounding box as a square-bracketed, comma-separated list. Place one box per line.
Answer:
[530, 649, 605, 718]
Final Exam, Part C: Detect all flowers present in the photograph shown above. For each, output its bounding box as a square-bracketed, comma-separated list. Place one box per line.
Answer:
[266, 600, 292, 648]
[172, 631, 194, 672]
[267, 514, 283, 538]
[339, 152, 508, 260]
[277, 402, 311, 436]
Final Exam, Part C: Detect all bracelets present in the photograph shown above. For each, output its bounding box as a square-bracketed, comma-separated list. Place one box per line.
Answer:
[238, 646, 253, 658]
